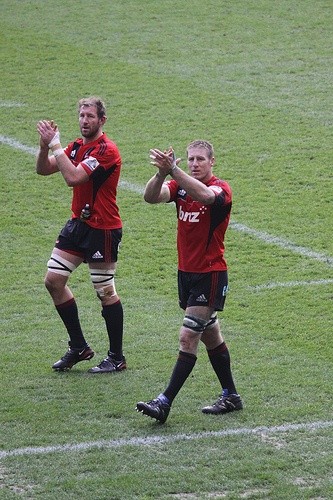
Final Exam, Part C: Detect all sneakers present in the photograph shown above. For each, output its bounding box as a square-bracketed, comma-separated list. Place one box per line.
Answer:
[51, 341, 95, 372]
[135, 395, 169, 423]
[88, 351, 126, 373]
[202, 389, 244, 415]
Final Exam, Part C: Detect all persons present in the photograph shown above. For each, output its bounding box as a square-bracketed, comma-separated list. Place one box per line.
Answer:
[35, 96, 129, 373]
[134, 139, 242, 424]
